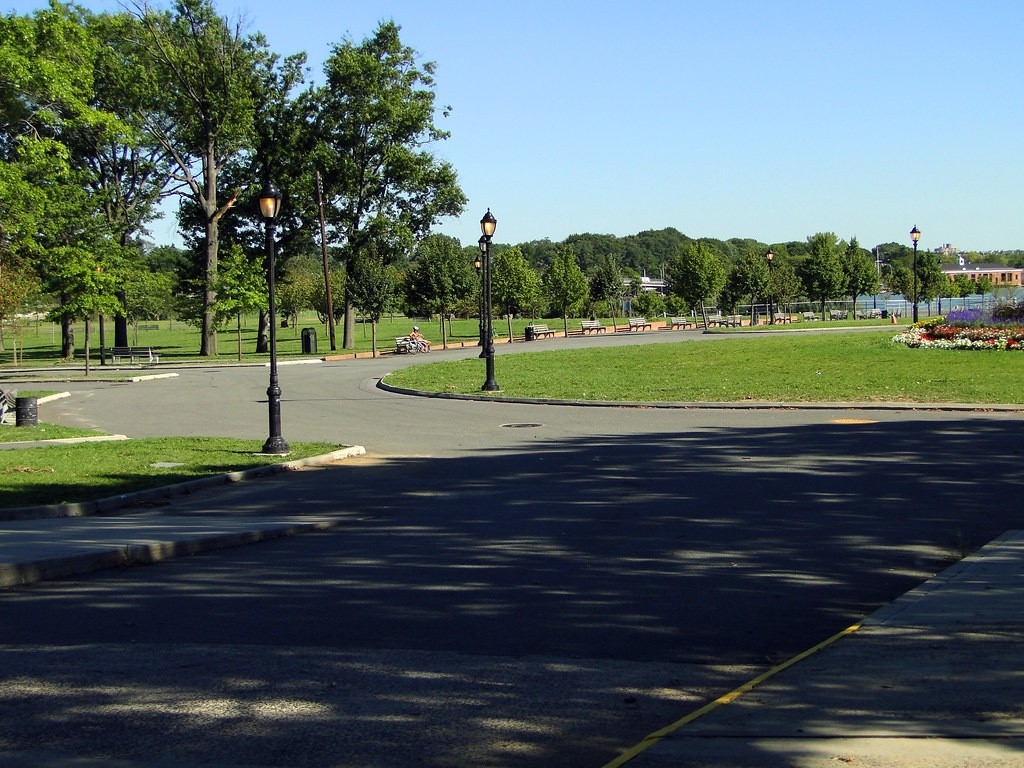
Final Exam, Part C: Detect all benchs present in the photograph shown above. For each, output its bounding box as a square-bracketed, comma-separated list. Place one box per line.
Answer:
[394, 336, 431, 355]
[853, 310, 868, 319]
[581, 321, 607, 336]
[719, 315, 742, 328]
[139, 325, 159, 332]
[671, 317, 692, 329]
[830, 310, 849, 321]
[707, 315, 724, 328]
[803, 312, 820, 322]
[775, 313, 791, 324]
[871, 308, 882, 318]
[628, 317, 652, 332]
[110, 347, 162, 366]
[531, 324, 556, 340]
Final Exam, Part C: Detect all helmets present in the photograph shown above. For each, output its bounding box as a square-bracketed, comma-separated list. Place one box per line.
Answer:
[413, 326, 419, 330]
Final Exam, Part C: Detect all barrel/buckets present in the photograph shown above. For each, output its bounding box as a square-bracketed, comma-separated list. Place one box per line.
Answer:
[15, 397, 38, 426]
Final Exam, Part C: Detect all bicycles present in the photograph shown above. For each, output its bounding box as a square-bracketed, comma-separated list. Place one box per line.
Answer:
[401, 334, 429, 355]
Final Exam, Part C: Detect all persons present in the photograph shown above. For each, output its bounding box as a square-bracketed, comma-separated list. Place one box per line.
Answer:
[409, 325, 431, 353]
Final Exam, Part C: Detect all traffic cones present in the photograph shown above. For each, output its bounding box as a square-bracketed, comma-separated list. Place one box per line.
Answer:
[891, 314, 897, 324]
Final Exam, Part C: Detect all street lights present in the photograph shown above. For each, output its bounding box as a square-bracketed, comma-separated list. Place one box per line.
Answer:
[910, 224, 921, 324]
[766, 249, 776, 325]
[252, 174, 291, 453]
[477, 235, 490, 359]
[479, 207, 499, 392]
[473, 254, 484, 347]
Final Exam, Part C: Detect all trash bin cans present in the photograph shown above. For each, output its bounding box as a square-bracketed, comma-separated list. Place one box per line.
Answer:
[300, 327, 318, 354]
[525, 326, 534, 341]
[15, 396, 38, 427]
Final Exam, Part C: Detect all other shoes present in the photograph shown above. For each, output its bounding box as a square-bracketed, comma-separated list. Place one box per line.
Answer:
[421, 350, 425, 353]
